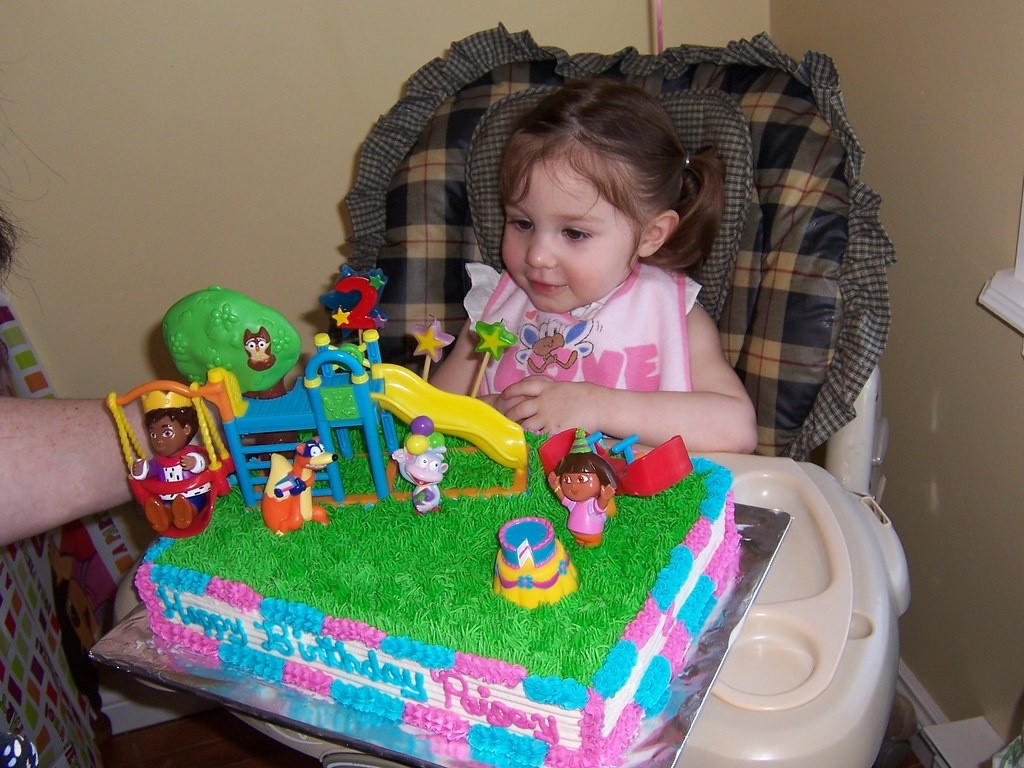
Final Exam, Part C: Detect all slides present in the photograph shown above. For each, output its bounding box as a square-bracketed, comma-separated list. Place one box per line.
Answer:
[371, 361, 527, 471]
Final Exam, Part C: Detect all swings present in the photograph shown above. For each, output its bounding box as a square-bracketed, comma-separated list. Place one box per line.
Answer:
[105, 383, 235, 540]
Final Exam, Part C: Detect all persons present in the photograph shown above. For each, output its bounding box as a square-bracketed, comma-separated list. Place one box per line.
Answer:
[546, 453, 621, 550]
[0, 212, 217, 768]
[425, 75, 758, 456]
[130, 385, 210, 532]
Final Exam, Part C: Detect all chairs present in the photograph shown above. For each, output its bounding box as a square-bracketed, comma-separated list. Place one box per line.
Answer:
[111, 20, 910, 767]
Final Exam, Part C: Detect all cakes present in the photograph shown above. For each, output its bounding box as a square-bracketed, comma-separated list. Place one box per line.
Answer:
[134, 418, 741, 768]
